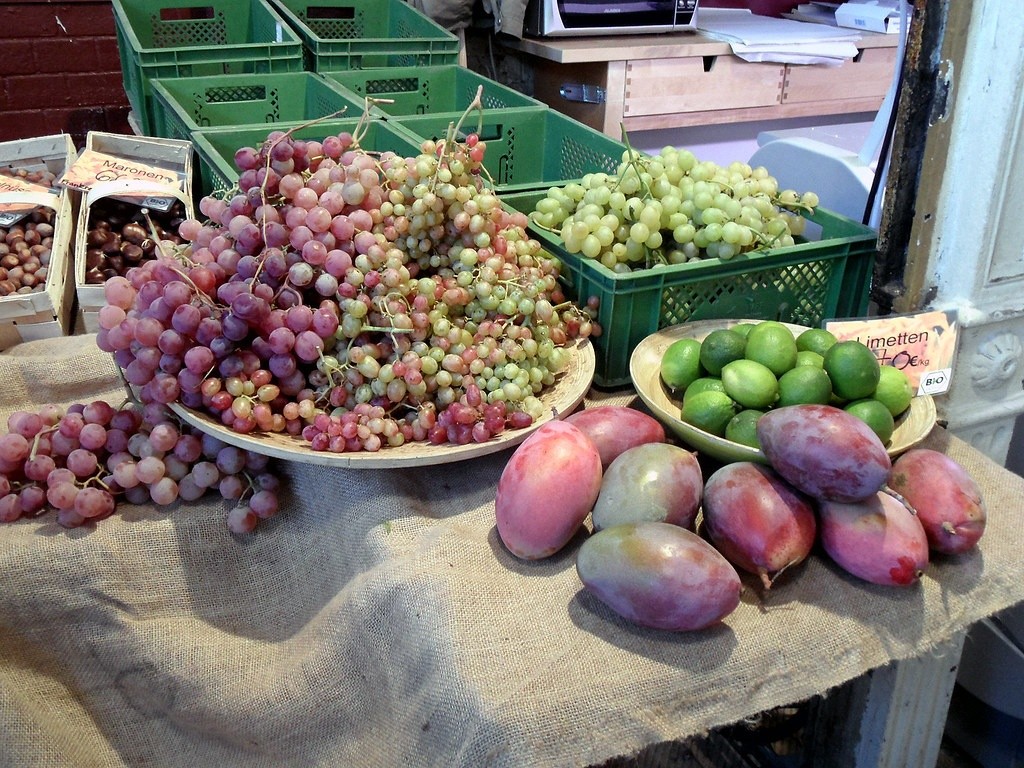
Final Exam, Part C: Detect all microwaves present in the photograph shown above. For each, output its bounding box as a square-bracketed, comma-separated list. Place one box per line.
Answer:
[522, 0, 698, 40]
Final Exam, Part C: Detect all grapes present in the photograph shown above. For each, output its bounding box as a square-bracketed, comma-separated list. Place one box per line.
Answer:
[529, 145, 826, 322]
[93, 132, 601, 453]
[0, 399, 280, 534]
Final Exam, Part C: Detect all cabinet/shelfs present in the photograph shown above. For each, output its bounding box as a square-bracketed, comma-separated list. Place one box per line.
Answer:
[500, 33, 899, 148]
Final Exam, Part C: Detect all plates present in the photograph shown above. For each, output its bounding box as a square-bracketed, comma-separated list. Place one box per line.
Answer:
[167, 335, 595, 469]
[630, 319, 937, 469]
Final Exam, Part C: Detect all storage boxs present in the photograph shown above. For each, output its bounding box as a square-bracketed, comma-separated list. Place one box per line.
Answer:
[497, 177, 879, 388]
[387, 108, 653, 190]
[320, 64, 549, 141]
[189, 121, 496, 204]
[145, 71, 382, 142]
[272, 0, 460, 93]
[0, 130, 194, 350]
[112, 0, 305, 137]
[836, 3, 911, 34]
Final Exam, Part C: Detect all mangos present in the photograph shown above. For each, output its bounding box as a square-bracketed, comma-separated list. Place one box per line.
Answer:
[494, 407, 988, 630]
[659, 319, 914, 449]
